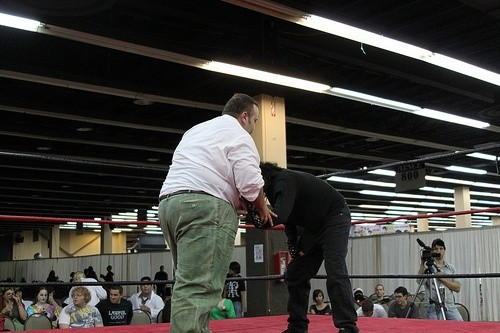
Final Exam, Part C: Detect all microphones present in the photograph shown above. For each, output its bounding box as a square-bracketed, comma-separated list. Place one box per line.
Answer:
[417, 239, 428, 249]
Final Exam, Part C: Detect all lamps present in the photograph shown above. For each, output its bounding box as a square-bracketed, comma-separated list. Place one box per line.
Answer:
[0, 0, 500, 233]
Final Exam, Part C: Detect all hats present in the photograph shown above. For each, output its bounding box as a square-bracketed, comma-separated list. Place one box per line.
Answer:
[432, 238, 445, 249]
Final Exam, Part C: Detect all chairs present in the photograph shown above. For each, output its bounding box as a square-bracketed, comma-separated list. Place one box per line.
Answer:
[1, 295, 163, 332]
[324, 290, 470, 321]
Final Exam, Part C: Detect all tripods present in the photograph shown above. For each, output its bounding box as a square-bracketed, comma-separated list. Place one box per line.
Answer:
[405, 268, 446, 320]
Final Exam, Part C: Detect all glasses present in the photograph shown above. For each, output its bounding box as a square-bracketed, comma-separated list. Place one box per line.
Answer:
[395, 296, 403, 298]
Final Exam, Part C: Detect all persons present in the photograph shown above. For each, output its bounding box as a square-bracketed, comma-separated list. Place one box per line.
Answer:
[220, 261, 247, 318]
[58, 287, 104, 329]
[237, 161, 359, 333]
[45, 269, 60, 281]
[153, 265, 168, 304]
[0, 285, 26, 333]
[128, 276, 165, 324]
[416, 239, 464, 322]
[155, 92, 277, 333]
[94, 284, 134, 326]
[83, 266, 99, 282]
[355, 300, 387, 318]
[63, 271, 108, 308]
[354, 285, 365, 300]
[308, 289, 332, 315]
[387, 286, 420, 319]
[100, 265, 115, 283]
[26, 286, 62, 330]
[372, 283, 387, 300]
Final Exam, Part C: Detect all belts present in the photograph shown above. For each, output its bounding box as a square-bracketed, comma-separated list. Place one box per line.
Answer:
[159, 190, 213, 201]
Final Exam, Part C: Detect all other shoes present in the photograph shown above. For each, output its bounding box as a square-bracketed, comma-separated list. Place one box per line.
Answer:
[338, 324, 359, 333]
[281, 327, 309, 333]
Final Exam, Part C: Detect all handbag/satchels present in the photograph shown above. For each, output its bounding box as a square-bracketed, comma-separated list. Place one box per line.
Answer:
[13, 319, 24, 331]
